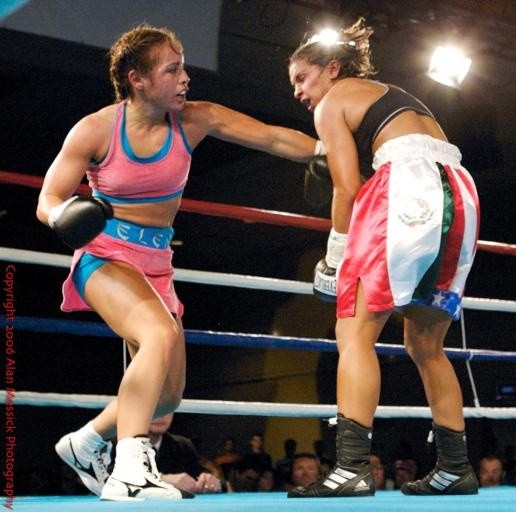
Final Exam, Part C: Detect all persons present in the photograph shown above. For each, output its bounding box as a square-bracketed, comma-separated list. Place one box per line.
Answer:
[32, 432, 516, 497]
[33, 24, 331, 502]
[287, 14, 483, 499]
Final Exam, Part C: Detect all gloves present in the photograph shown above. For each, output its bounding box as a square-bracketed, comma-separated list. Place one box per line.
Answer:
[47, 196, 113, 249]
[314, 260, 339, 304]
[303, 155, 333, 207]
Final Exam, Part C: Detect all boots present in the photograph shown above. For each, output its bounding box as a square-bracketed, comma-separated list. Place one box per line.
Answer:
[401, 423, 478, 495]
[101, 438, 182, 502]
[287, 413, 375, 497]
[55, 423, 113, 495]
